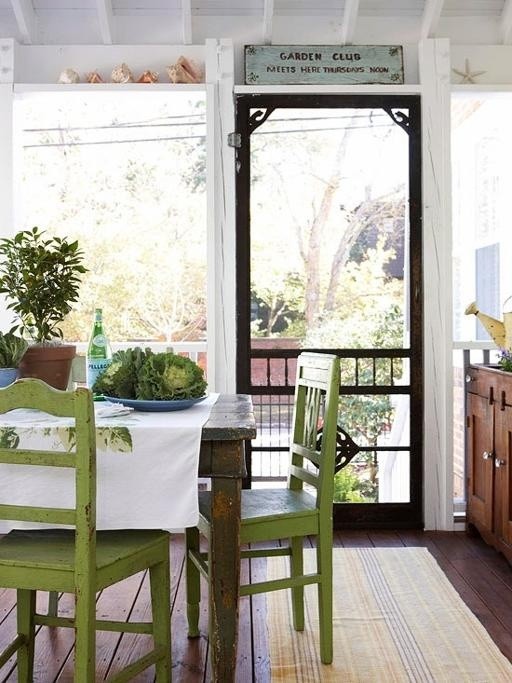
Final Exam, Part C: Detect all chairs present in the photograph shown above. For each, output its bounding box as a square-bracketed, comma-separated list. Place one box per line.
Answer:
[0, 376, 172, 682]
[185, 350, 343, 667]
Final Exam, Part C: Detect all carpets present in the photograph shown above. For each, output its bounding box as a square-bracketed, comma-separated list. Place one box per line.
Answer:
[265, 544, 510, 682]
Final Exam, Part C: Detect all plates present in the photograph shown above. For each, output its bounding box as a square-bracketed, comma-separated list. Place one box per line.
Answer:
[107, 393, 210, 413]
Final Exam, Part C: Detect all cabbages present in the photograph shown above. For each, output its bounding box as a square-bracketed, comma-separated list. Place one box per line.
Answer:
[91, 346, 208, 401]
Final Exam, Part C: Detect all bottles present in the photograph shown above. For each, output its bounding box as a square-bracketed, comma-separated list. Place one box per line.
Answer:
[85, 310, 113, 401]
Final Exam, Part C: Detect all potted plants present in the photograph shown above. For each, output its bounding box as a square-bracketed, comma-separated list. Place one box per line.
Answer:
[0, 225, 90, 391]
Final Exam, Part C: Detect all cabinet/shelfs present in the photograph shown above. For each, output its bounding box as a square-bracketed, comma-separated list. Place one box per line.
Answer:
[463, 361, 512, 564]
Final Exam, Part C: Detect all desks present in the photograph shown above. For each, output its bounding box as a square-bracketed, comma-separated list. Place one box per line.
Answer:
[0, 392, 257, 683]
